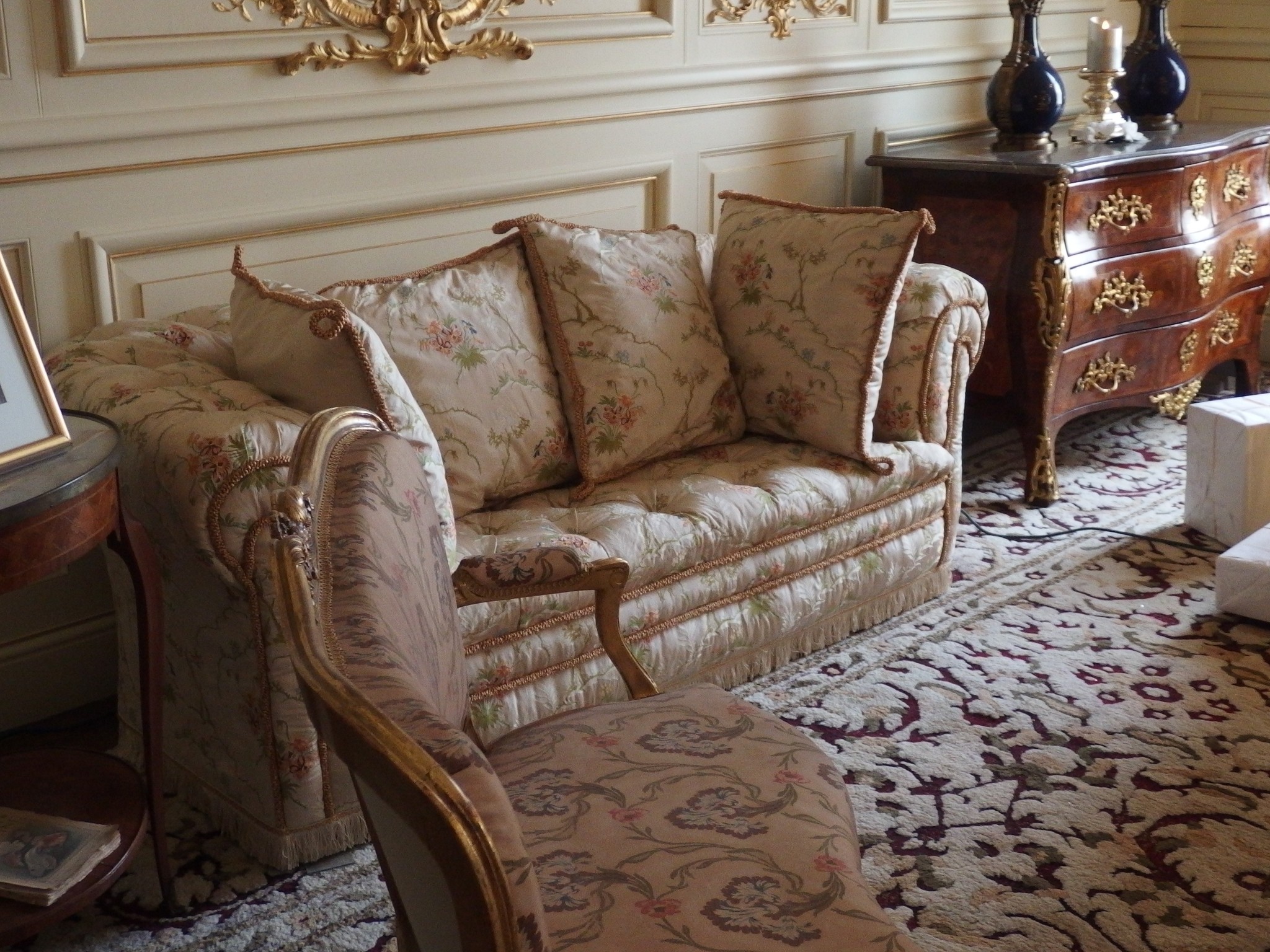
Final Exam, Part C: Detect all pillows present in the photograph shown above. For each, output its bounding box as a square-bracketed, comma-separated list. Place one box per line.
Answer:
[316, 231, 582, 521]
[231, 243, 456, 573]
[708, 188, 937, 476]
[490, 210, 750, 501]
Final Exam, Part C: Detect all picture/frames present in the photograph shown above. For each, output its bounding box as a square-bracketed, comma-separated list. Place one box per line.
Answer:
[1, 249, 74, 477]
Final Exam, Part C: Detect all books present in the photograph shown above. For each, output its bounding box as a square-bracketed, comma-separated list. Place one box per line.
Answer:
[0, 805, 122, 906]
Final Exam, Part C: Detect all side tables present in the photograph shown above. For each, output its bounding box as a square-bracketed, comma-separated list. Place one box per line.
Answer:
[0, 405, 182, 952]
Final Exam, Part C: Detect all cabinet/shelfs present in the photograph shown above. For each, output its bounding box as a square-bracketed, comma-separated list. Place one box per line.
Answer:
[865, 118, 1270, 507]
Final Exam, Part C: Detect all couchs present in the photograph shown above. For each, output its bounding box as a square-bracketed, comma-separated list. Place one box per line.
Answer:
[45, 231, 993, 871]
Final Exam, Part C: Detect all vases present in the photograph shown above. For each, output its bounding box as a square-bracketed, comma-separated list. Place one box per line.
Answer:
[983, 0, 1066, 154]
[1112, 0, 1191, 131]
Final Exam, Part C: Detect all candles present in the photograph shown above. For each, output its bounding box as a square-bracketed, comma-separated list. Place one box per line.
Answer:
[1087, 16, 1124, 72]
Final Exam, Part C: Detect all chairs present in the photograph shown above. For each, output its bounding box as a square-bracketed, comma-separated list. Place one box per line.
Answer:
[271, 406, 928, 951]
